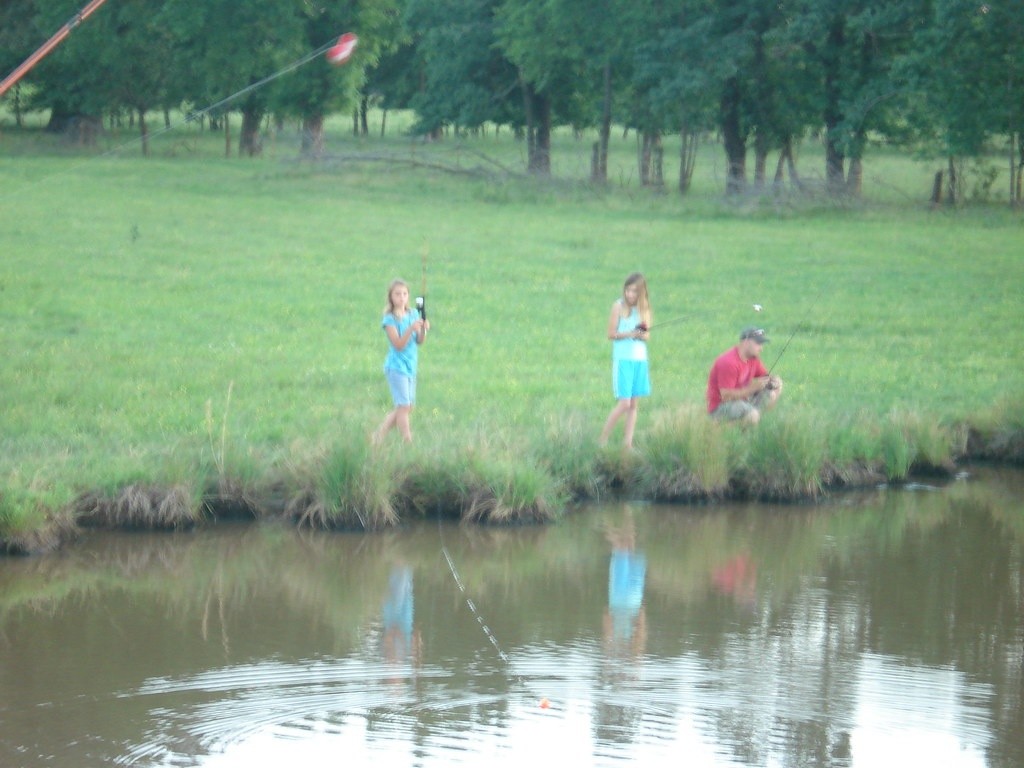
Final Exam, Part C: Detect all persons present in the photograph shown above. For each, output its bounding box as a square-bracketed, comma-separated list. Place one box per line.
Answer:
[602, 514, 647, 668]
[382, 558, 422, 687]
[372, 279, 430, 448]
[706, 327, 782, 427]
[599, 272, 651, 449]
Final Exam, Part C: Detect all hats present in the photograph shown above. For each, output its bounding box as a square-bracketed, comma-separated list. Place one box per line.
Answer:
[740, 328, 769, 343]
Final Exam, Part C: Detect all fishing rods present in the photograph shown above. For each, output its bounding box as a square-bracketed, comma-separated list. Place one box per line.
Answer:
[626, 300, 767, 342]
[412, 239, 428, 327]
[745, 304, 827, 405]
[0, 0, 365, 216]
